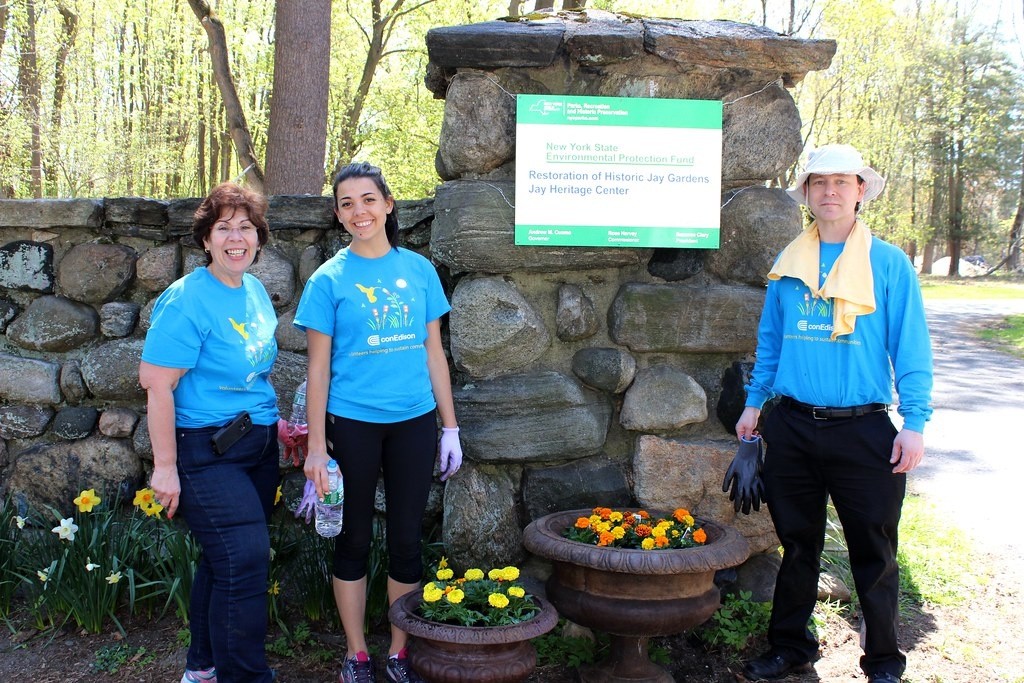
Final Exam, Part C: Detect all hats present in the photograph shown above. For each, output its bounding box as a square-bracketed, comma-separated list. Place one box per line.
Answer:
[785, 145, 885, 204]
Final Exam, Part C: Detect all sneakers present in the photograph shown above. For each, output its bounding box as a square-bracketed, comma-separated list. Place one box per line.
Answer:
[180, 667, 218, 683]
[383, 655, 424, 683]
[339, 653, 375, 682]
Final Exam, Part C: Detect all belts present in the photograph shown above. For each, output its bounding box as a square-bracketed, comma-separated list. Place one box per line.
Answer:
[779, 396, 887, 420]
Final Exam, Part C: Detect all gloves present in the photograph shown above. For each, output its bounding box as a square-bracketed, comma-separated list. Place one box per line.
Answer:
[439, 426, 463, 481]
[722, 436, 768, 514]
[276, 419, 309, 466]
[295, 481, 321, 525]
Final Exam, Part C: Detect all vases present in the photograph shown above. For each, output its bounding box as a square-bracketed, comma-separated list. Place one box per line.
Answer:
[388, 587, 558, 683]
[523, 509, 750, 683]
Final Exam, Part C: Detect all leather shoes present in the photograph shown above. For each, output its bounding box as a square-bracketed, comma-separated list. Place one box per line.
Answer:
[869, 672, 900, 683]
[743, 651, 811, 680]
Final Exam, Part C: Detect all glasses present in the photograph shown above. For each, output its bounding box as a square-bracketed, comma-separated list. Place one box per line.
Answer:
[210, 224, 258, 237]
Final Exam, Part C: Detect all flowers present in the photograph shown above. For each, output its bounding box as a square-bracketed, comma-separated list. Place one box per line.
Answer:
[416, 556, 542, 626]
[560, 506, 707, 550]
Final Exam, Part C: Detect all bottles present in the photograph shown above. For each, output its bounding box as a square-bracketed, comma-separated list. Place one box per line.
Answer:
[288, 376, 307, 427]
[315, 460, 344, 537]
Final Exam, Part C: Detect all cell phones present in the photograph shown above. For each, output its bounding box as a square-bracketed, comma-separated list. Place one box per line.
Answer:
[211, 411, 253, 456]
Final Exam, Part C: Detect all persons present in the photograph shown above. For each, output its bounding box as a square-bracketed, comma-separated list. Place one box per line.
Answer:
[139, 183, 280, 683]
[292, 161, 463, 683]
[735, 146, 934, 682]
